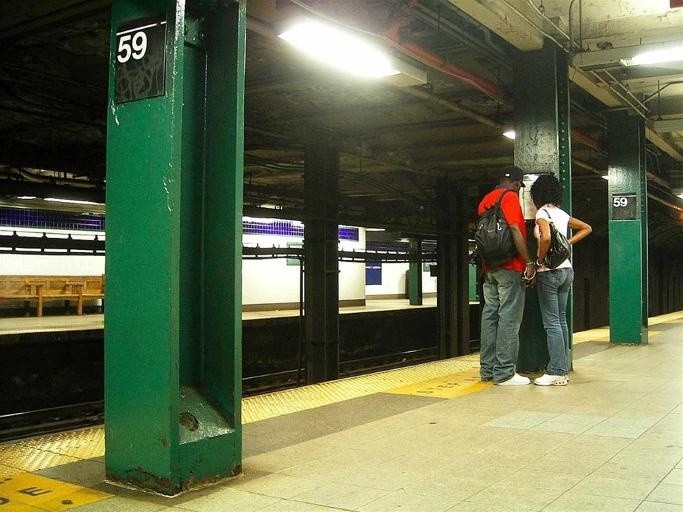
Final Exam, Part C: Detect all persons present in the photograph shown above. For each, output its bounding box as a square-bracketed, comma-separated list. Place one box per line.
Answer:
[529, 175, 591, 385]
[474, 165, 538, 387]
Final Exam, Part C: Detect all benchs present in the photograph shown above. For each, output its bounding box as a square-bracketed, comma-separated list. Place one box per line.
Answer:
[0, 274, 104, 317]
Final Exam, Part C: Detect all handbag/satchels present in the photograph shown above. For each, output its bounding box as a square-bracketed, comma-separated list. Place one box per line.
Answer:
[543, 209, 571, 269]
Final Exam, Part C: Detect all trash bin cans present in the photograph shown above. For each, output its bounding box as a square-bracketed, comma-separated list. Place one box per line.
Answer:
[405, 270, 410, 299]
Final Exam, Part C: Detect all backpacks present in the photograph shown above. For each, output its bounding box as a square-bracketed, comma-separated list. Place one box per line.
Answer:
[474, 190, 517, 264]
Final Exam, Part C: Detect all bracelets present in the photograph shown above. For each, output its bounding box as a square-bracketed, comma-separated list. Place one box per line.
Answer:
[536, 259, 543, 267]
[524, 267, 536, 280]
[525, 261, 536, 266]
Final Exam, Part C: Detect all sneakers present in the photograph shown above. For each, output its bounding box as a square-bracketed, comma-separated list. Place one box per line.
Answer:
[533, 374, 570, 385]
[499, 373, 530, 385]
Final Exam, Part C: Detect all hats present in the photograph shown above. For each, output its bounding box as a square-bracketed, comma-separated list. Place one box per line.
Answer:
[503, 166, 525, 187]
